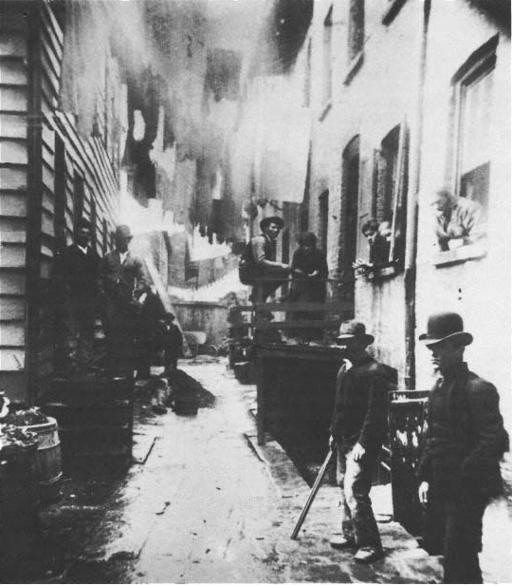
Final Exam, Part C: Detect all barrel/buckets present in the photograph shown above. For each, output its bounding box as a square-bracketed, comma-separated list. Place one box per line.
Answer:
[0, 416, 66, 584]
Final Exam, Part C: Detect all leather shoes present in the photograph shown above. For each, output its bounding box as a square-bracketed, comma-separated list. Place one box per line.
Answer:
[329, 537, 351, 546]
[354, 547, 383, 560]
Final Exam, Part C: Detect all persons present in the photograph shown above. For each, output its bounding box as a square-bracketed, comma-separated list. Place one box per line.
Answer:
[415, 312, 507, 583]
[429, 188, 483, 241]
[235, 211, 332, 347]
[326, 318, 396, 565]
[56, 218, 182, 381]
[350, 217, 408, 278]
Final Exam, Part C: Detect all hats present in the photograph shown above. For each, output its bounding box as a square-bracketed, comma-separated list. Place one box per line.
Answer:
[260, 206, 283, 230]
[419, 313, 472, 345]
[335, 321, 374, 345]
[111, 225, 132, 241]
